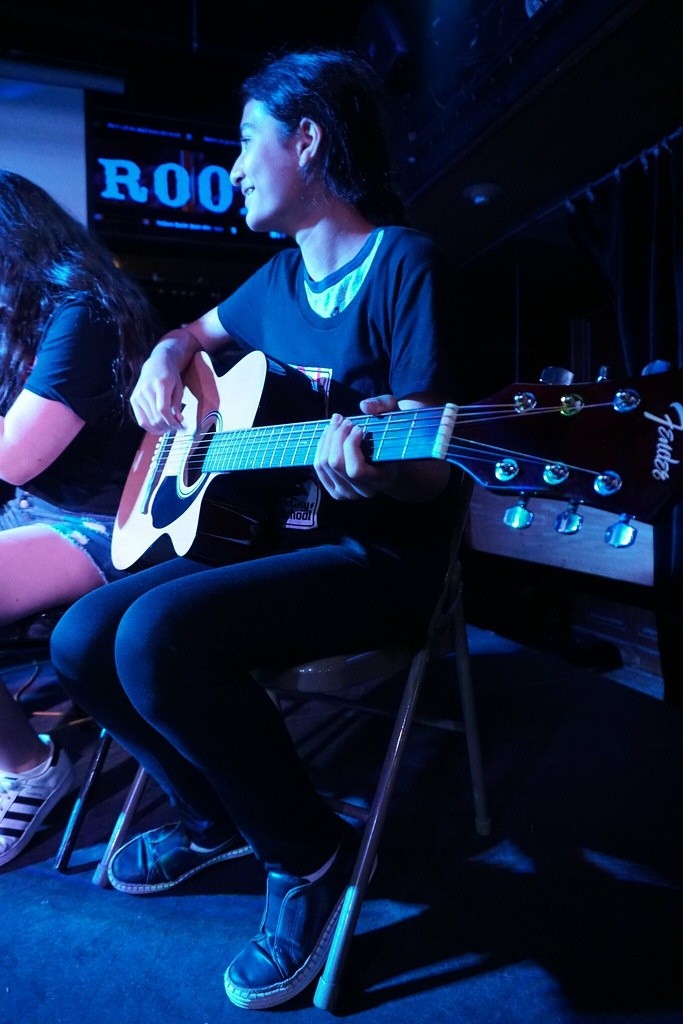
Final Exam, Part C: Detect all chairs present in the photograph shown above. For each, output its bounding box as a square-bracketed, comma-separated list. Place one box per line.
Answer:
[16, 461, 506, 1014]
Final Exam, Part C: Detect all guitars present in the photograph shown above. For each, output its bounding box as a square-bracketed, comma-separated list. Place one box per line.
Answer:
[105, 343, 683, 567]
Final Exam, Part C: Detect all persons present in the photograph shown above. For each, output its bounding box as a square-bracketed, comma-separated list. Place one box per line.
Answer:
[0, 169, 165, 865]
[49, 55, 473, 1010]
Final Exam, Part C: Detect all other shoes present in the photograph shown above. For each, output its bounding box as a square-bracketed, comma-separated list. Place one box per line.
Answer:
[108, 820, 255, 894]
[224, 820, 378, 1009]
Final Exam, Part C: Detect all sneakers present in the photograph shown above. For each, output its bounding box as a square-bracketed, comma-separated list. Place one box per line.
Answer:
[0, 734, 74, 870]
